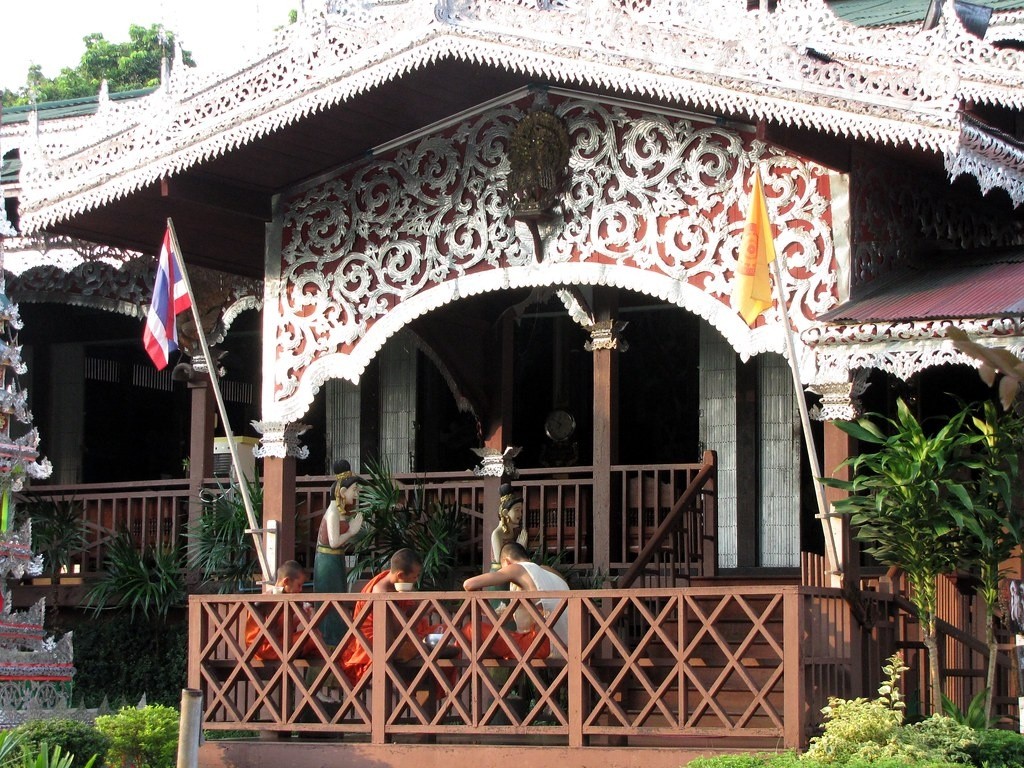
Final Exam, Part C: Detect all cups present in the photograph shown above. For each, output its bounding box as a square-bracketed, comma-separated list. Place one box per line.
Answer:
[272, 587, 284, 594]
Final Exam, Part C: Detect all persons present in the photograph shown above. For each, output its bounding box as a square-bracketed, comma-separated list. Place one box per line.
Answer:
[453, 541, 571, 659]
[311, 460, 364, 646]
[338, 547, 439, 686]
[243, 561, 333, 660]
[483, 482, 527, 685]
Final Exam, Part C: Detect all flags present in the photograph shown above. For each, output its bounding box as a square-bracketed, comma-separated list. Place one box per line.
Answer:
[735, 168, 779, 329]
[143, 225, 193, 372]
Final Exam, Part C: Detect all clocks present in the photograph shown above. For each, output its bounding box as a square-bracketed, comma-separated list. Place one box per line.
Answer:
[543, 408, 575, 441]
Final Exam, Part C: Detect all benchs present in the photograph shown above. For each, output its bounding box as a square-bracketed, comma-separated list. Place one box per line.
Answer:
[210, 655, 596, 725]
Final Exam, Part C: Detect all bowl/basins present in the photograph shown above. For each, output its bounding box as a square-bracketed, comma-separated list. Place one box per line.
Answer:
[428, 634, 454, 645]
[394, 583, 414, 592]
